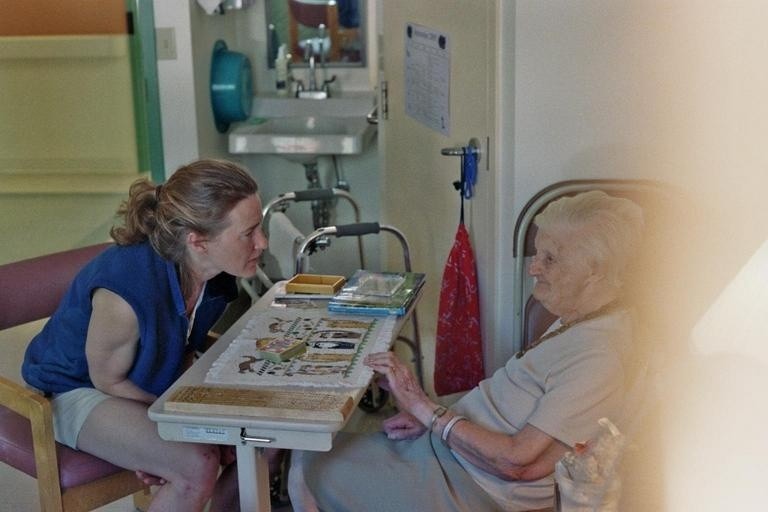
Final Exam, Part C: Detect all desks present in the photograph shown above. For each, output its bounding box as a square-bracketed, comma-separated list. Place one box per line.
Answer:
[147, 281, 423, 512]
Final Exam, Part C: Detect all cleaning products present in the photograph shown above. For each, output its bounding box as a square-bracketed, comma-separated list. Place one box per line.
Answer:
[275, 45, 289, 95]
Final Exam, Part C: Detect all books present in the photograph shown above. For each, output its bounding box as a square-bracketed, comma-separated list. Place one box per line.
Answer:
[273, 268, 428, 317]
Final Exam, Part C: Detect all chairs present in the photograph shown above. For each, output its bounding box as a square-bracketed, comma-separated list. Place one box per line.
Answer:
[513, 178, 682, 355]
[288, 0, 359, 63]
[0, 242, 152, 512]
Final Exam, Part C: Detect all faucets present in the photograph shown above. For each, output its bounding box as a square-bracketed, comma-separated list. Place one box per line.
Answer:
[304, 40, 312, 59]
[309, 57, 316, 91]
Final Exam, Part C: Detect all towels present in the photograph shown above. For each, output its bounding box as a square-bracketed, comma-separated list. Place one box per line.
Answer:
[268, 211, 310, 281]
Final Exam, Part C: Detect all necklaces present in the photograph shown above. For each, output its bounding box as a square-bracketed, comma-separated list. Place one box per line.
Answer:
[514, 300, 623, 359]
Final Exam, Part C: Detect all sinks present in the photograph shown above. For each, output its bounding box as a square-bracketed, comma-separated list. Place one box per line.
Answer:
[252, 117, 357, 135]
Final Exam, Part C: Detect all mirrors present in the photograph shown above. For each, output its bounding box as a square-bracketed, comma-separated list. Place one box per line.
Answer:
[264, 0, 366, 68]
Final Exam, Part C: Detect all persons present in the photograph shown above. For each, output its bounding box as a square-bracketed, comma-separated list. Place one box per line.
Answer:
[19, 159, 285, 512]
[336, 0, 361, 61]
[286, 190, 643, 512]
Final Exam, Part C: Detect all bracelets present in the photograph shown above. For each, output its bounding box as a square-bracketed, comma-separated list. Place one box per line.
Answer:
[441, 415, 471, 450]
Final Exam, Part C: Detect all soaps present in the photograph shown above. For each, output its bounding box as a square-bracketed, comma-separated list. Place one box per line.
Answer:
[250, 116, 262, 124]
